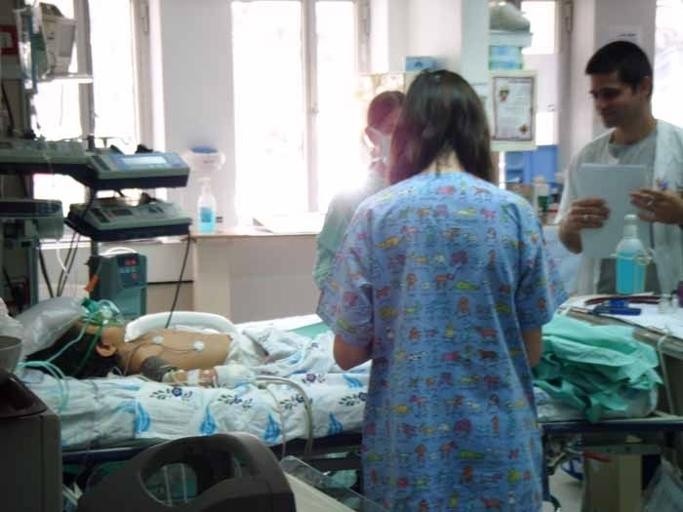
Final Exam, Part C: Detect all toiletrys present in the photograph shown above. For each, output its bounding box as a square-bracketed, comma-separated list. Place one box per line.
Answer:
[196, 177, 216, 231]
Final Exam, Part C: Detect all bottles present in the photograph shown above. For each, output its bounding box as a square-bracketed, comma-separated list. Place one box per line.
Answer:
[615, 213, 647, 296]
[214, 215, 224, 234]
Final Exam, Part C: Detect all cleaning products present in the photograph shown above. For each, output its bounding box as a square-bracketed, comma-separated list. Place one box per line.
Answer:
[616, 211, 651, 296]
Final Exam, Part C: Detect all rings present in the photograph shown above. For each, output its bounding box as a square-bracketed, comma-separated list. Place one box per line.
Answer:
[584, 214, 588, 222]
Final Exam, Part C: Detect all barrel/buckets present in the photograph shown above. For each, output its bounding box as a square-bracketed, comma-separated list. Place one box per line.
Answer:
[490, 46, 525, 71]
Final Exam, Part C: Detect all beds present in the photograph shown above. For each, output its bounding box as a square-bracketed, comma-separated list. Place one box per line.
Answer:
[9, 323, 683, 507]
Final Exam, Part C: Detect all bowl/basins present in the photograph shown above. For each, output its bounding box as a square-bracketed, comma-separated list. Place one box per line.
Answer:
[0, 335, 23, 377]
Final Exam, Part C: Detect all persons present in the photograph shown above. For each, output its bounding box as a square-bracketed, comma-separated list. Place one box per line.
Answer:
[312, 90, 405, 292]
[551, 41, 683, 500]
[26, 319, 261, 387]
[315, 71, 570, 512]
